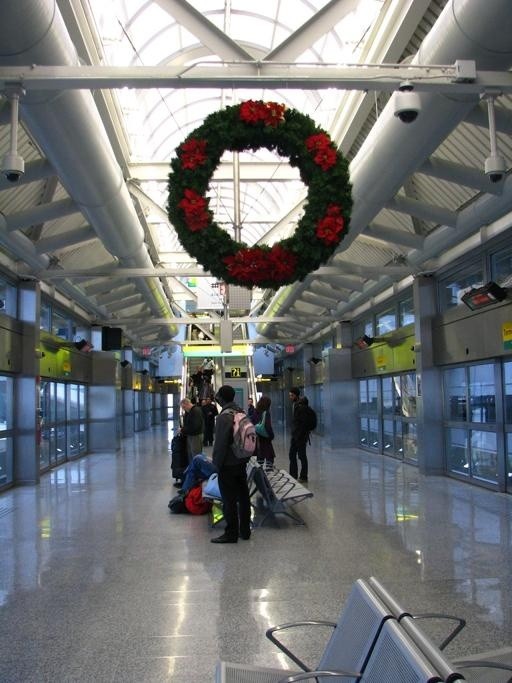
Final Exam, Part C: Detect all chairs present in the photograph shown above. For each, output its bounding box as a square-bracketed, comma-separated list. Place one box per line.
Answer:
[216, 576, 512, 683]
[201, 456, 313, 527]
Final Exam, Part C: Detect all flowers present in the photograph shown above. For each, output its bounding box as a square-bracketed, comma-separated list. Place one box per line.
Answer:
[167, 99, 354, 292]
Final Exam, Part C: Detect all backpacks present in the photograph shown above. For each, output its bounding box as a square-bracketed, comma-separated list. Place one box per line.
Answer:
[307, 404, 317, 432]
[222, 408, 256, 458]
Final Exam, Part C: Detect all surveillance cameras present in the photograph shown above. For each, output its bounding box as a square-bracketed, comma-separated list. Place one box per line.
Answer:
[1, 156, 25, 183]
[485, 157, 506, 183]
[393, 92, 422, 124]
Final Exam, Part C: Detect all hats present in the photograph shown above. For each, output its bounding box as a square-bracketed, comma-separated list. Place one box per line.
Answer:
[291, 388, 299, 396]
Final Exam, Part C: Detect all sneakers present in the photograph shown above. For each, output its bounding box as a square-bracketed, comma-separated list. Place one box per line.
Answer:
[299, 478, 307, 482]
[173, 484, 185, 494]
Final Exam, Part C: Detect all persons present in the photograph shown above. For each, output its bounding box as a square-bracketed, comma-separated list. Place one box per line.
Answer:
[251, 396, 277, 473]
[186, 357, 218, 447]
[211, 385, 251, 543]
[287, 387, 308, 483]
[201, 312, 213, 341]
[246, 397, 254, 416]
[178, 454, 217, 494]
[176, 398, 205, 461]
[189, 312, 200, 340]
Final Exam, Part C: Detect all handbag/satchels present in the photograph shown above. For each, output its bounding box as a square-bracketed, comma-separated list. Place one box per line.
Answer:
[168, 483, 213, 515]
[255, 410, 271, 438]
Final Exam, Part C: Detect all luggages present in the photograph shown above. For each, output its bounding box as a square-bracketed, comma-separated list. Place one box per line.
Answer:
[171, 432, 188, 480]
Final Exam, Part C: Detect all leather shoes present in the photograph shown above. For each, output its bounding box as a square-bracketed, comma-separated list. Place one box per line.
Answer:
[212, 535, 236, 543]
